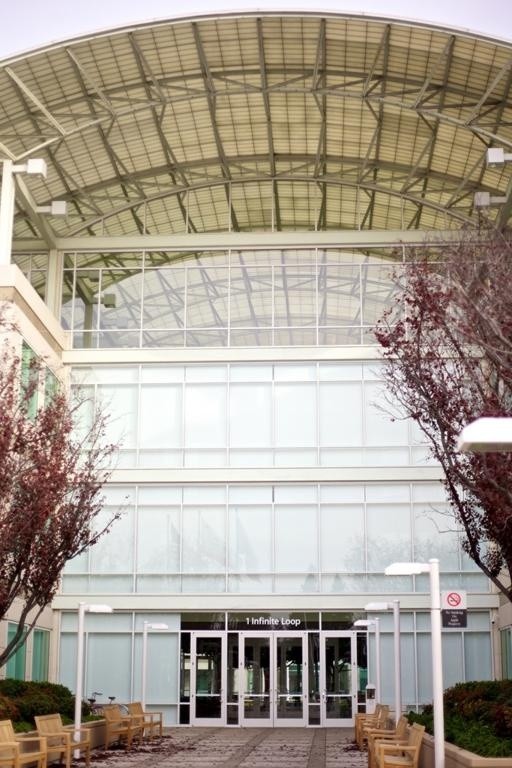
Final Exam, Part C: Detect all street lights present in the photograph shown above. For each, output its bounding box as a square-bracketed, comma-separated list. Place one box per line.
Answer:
[458, 417, 512, 454]
[74, 603, 113, 759]
[141, 620, 168, 712]
[364, 600, 402, 727]
[384, 558, 445, 768]
[353, 617, 381, 705]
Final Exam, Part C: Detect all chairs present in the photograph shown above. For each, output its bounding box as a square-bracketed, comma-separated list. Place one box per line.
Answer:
[0, 702, 163, 768]
[354, 703, 426, 768]
